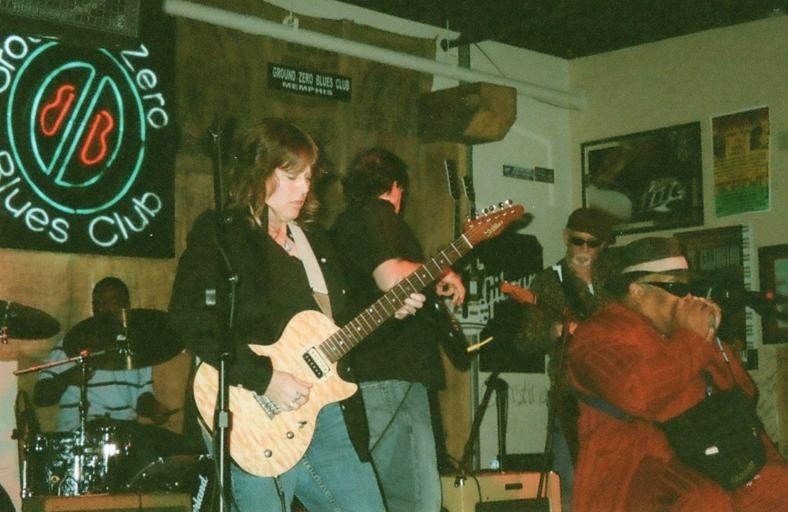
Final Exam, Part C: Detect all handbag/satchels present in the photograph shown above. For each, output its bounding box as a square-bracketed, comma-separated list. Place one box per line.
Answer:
[659, 384, 765, 492]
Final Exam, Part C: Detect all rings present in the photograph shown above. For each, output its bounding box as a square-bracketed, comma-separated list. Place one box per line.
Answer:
[294, 392, 302, 399]
[287, 403, 294, 409]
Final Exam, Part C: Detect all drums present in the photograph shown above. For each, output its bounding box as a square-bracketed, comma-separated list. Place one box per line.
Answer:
[126, 454, 228, 512]
[23, 427, 140, 497]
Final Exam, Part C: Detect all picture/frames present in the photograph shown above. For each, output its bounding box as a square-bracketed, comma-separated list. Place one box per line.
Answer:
[581, 121, 704, 236]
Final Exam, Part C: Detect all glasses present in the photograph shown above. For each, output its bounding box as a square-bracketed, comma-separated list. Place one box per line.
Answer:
[568, 233, 602, 247]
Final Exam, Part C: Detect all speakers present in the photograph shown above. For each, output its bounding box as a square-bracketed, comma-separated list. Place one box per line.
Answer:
[419, 82, 517, 145]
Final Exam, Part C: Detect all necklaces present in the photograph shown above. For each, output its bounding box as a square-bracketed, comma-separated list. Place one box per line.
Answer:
[259, 220, 293, 252]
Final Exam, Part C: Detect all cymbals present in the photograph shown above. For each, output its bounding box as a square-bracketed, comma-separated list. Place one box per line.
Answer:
[1, 300, 61, 339]
[62, 308, 184, 370]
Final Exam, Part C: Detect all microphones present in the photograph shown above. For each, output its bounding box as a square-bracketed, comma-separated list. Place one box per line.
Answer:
[705, 285, 784, 307]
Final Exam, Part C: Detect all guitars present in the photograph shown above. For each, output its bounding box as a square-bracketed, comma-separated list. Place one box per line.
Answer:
[191, 199, 523, 477]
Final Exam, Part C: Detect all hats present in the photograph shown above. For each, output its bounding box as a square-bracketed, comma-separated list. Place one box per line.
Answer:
[601, 233, 701, 290]
[566, 207, 614, 241]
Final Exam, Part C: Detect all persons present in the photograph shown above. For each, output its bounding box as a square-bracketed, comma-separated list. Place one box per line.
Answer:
[569, 236, 788, 512]
[171, 117, 388, 510]
[328, 149, 466, 511]
[517, 209, 623, 506]
[32, 278, 172, 430]
